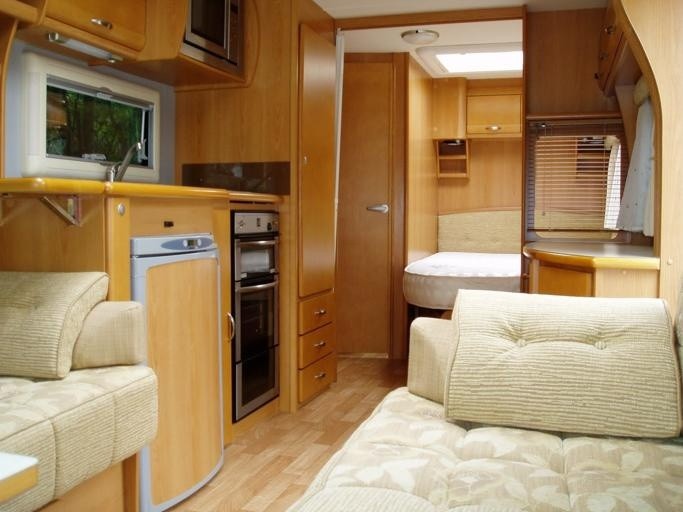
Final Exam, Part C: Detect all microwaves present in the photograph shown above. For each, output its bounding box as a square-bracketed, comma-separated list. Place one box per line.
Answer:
[179, 0, 243, 78]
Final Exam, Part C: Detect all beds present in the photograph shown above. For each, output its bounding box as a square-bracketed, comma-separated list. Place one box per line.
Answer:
[401, 203, 526, 314]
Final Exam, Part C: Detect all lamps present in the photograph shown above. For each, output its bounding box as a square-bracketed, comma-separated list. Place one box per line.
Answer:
[402, 30, 439, 46]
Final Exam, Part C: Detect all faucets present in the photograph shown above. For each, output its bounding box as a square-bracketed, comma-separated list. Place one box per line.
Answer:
[99, 142, 143, 181]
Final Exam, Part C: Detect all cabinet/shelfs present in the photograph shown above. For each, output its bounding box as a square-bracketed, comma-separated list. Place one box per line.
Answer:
[279, 21, 345, 408]
[536, 265, 660, 299]
[431, 76, 522, 183]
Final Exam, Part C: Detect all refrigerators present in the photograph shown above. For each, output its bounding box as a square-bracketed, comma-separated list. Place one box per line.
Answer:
[130, 233, 224, 512]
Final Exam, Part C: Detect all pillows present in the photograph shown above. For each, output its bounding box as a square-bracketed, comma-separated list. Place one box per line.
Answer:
[446, 285, 679, 436]
[3, 268, 110, 379]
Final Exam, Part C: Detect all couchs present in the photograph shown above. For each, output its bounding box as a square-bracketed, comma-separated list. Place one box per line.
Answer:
[281, 269, 683, 511]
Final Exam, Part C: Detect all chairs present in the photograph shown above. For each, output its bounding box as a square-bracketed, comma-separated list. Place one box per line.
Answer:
[1, 263, 163, 512]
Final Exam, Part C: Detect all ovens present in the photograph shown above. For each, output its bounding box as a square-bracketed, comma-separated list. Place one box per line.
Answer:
[231, 210, 282, 426]
